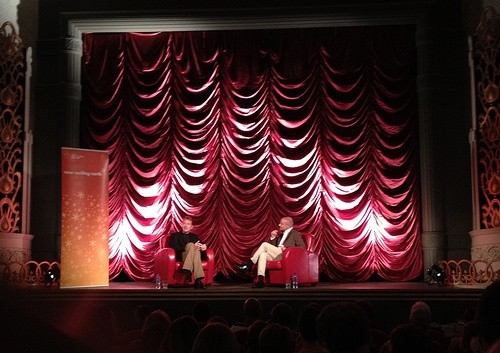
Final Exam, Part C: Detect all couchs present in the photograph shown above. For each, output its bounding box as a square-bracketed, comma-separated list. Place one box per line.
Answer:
[153, 235, 215, 288]
[258, 232, 319, 287]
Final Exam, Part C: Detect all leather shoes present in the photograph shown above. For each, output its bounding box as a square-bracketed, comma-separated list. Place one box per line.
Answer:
[236, 263, 252, 273]
[251, 282, 265, 288]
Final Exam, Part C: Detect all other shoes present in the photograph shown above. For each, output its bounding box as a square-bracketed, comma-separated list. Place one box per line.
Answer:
[178, 269, 191, 282]
[194, 280, 204, 289]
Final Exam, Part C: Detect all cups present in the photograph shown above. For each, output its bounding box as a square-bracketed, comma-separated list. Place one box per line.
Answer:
[286, 280, 291, 289]
[162, 278, 168, 289]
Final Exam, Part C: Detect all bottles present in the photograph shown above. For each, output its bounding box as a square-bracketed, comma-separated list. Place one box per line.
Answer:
[155, 274, 161, 289]
[292, 272, 298, 289]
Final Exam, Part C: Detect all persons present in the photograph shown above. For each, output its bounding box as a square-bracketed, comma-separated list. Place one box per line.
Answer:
[232, 216, 305, 288]
[127, 299, 500, 353]
[169, 216, 206, 289]
[478, 280, 500, 353]
